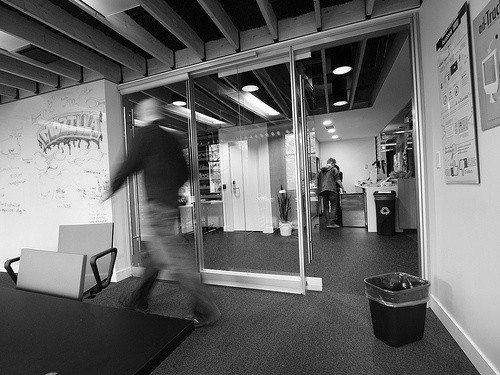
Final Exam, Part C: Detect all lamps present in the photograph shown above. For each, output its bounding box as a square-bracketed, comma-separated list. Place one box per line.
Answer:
[332, 73, 348, 106]
[331, 46, 353, 76]
[241, 71, 259, 91]
[171, 92, 187, 106]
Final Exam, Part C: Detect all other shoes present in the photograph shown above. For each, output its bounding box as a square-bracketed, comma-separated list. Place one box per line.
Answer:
[326, 224, 340, 228]
[119, 293, 149, 313]
[185, 314, 205, 328]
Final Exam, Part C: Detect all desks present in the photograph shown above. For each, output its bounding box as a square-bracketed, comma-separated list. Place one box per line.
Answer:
[0, 287, 197, 375]
[175, 199, 223, 241]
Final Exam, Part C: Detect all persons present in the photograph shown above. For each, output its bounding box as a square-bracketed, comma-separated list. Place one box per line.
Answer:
[335, 165, 342, 225]
[317, 158, 346, 228]
[98, 98, 220, 327]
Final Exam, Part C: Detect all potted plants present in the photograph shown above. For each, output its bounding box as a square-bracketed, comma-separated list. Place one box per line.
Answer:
[275, 192, 293, 237]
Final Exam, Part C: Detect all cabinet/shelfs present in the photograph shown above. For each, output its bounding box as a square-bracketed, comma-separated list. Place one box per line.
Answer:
[356, 184, 400, 232]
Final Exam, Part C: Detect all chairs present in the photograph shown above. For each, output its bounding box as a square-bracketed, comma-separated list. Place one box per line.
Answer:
[3, 221, 118, 302]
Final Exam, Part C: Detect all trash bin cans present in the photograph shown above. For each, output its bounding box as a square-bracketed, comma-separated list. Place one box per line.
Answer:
[364, 272, 431, 347]
[373, 190, 396, 235]
